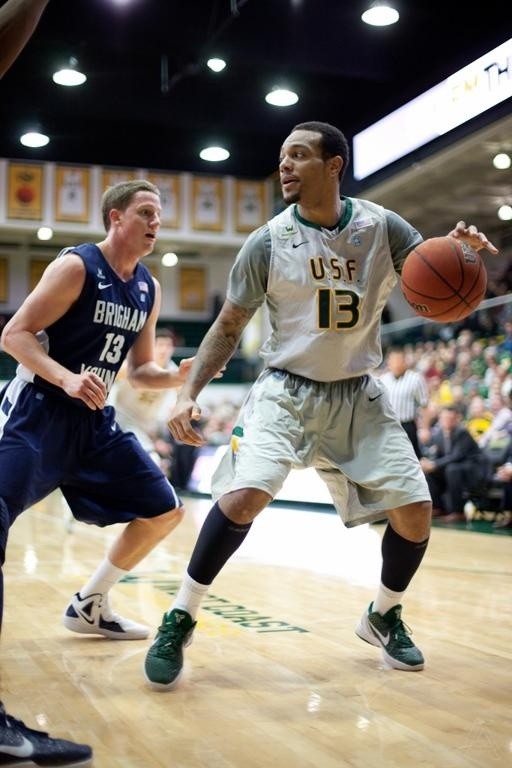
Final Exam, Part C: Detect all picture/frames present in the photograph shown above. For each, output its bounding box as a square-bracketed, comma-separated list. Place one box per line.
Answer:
[101, 169, 135, 191]
[54, 165, 89, 221]
[149, 171, 181, 228]
[235, 178, 268, 233]
[192, 175, 224, 230]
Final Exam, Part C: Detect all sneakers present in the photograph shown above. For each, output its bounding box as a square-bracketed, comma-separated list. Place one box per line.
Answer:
[143, 607, 197, 689]
[61, 592, 149, 639]
[355, 598, 427, 673]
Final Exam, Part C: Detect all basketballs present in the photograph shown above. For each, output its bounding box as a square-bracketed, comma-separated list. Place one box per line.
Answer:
[401, 237, 487, 321]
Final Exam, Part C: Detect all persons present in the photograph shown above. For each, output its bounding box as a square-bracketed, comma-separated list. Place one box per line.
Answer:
[106, 330, 236, 499]
[143, 122, 500, 690]
[1, 180, 226, 642]
[374, 266, 511, 523]
